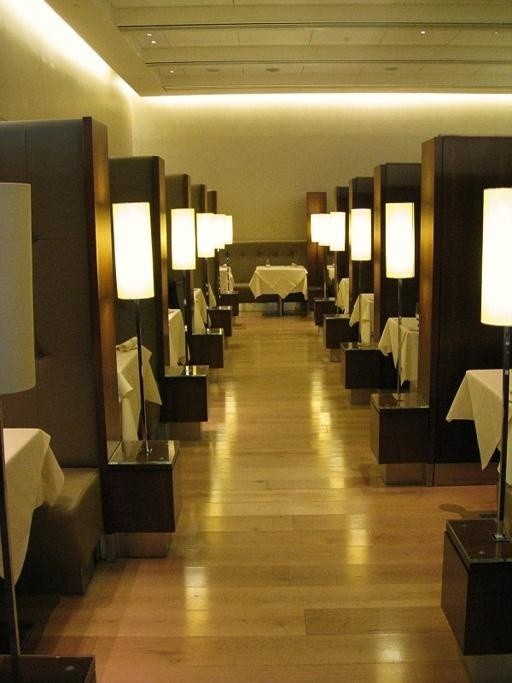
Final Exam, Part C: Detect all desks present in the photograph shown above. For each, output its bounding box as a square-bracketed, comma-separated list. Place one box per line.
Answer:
[164, 365, 209, 440]
[342, 342, 396, 404]
[314, 297, 335, 327]
[0, 654, 96, 683]
[370, 394, 428, 485]
[441, 520, 512, 654]
[107, 441, 181, 558]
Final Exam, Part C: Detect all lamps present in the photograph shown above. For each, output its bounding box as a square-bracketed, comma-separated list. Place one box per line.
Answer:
[225, 215, 233, 292]
[113, 202, 155, 454]
[480, 188, 512, 543]
[386, 201, 414, 401]
[310, 213, 319, 289]
[330, 212, 346, 317]
[0, 182, 36, 683]
[351, 208, 371, 345]
[319, 214, 330, 299]
[170, 208, 196, 372]
[196, 213, 215, 332]
[215, 214, 225, 308]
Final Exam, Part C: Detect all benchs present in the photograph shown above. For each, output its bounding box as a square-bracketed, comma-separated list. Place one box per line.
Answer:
[18, 467, 102, 596]
